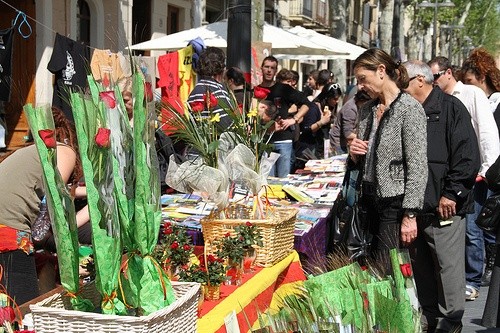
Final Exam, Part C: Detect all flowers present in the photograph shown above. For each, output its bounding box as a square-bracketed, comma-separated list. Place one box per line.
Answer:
[0, 60, 422, 333]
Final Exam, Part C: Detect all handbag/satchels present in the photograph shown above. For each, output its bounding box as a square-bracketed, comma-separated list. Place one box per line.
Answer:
[328, 155, 375, 261]
[475, 194, 500, 231]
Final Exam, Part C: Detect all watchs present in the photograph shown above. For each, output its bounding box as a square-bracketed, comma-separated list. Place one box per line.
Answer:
[404, 210, 418, 218]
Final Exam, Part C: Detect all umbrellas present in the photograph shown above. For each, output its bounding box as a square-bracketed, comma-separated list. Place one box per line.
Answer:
[124, 18, 367, 61]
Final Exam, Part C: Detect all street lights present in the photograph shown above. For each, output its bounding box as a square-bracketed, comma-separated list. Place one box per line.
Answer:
[438, 21, 465, 68]
[417, 0, 456, 59]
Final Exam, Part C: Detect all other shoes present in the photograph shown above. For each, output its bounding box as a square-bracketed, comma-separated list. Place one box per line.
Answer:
[465, 285, 479, 300]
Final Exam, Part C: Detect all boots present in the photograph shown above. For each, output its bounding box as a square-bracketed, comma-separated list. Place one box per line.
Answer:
[480, 242, 497, 286]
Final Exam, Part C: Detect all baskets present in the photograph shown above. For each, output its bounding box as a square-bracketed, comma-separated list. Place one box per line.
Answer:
[29, 277, 201, 333]
[201, 204, 299, 267]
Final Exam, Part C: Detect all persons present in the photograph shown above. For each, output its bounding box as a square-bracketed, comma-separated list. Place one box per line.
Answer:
[0, 106, 89, 310]
[186, 42, 500, 333]
[122, 78, 180, 195]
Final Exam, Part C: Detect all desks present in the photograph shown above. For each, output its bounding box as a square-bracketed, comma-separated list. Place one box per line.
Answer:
[155, 175, 345, 279]
[0, 245, 309, 333]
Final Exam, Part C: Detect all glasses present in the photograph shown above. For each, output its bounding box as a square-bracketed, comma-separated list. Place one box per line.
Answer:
[433, 66, 448, 80]
[328, 83, 341, 91]
